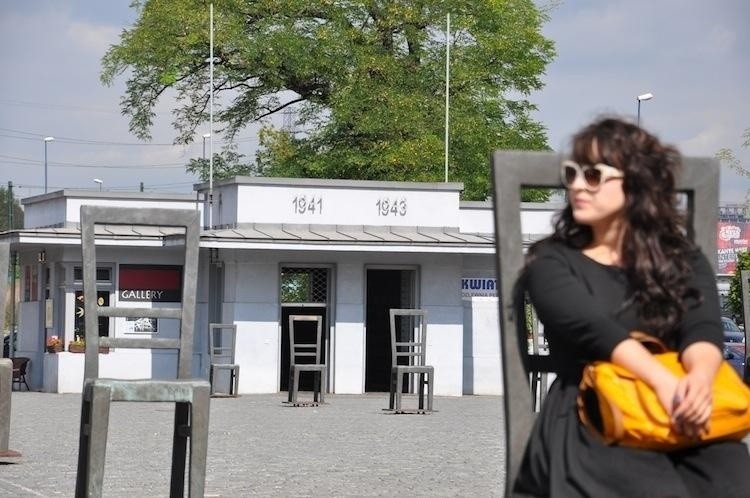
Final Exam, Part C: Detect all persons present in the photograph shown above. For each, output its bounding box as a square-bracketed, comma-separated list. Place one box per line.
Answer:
[506, 116, 750, 496]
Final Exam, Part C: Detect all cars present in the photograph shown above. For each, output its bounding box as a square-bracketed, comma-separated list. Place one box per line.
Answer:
[715, 272, 742, 304]
[720, 316, 745, 383]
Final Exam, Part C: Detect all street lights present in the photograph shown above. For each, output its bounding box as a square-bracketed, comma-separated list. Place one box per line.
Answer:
[200, 131, 212, 184]
[94, 177, 103, 190]
[44, 136, 53, 194]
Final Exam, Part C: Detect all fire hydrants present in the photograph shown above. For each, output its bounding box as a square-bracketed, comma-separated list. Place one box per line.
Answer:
[637, 91, 652, 130]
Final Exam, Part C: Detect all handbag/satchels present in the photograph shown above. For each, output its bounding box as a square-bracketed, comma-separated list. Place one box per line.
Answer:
[573, 329, 750, 452]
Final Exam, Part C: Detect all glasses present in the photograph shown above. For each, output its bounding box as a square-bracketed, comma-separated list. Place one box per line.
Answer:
[560, 161, 624, 191]
